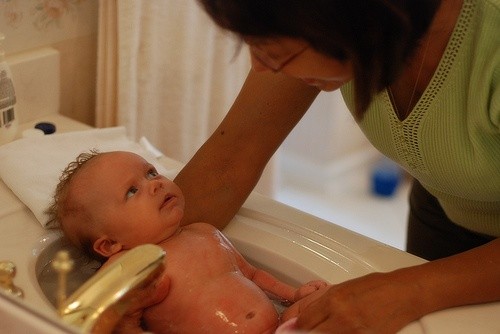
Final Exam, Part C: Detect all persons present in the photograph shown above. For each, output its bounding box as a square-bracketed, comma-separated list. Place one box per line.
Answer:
[168, 0, 500, 334]
[46, 149, 330, 334]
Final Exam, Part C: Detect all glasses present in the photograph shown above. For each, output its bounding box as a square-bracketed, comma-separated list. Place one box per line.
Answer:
[249, 44, 311, 73]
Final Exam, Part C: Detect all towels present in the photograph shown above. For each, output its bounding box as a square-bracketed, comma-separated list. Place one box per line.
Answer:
[0, 125, 169, 231]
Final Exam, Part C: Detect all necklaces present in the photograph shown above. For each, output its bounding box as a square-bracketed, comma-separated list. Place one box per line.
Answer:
[387, 29, 430, 143]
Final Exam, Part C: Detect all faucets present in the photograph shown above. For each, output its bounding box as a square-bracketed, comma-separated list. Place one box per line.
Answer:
[55, 242, 168, 334]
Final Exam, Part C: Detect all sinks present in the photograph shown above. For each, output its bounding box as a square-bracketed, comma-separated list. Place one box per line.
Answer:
[23, 205, 427, 334]
